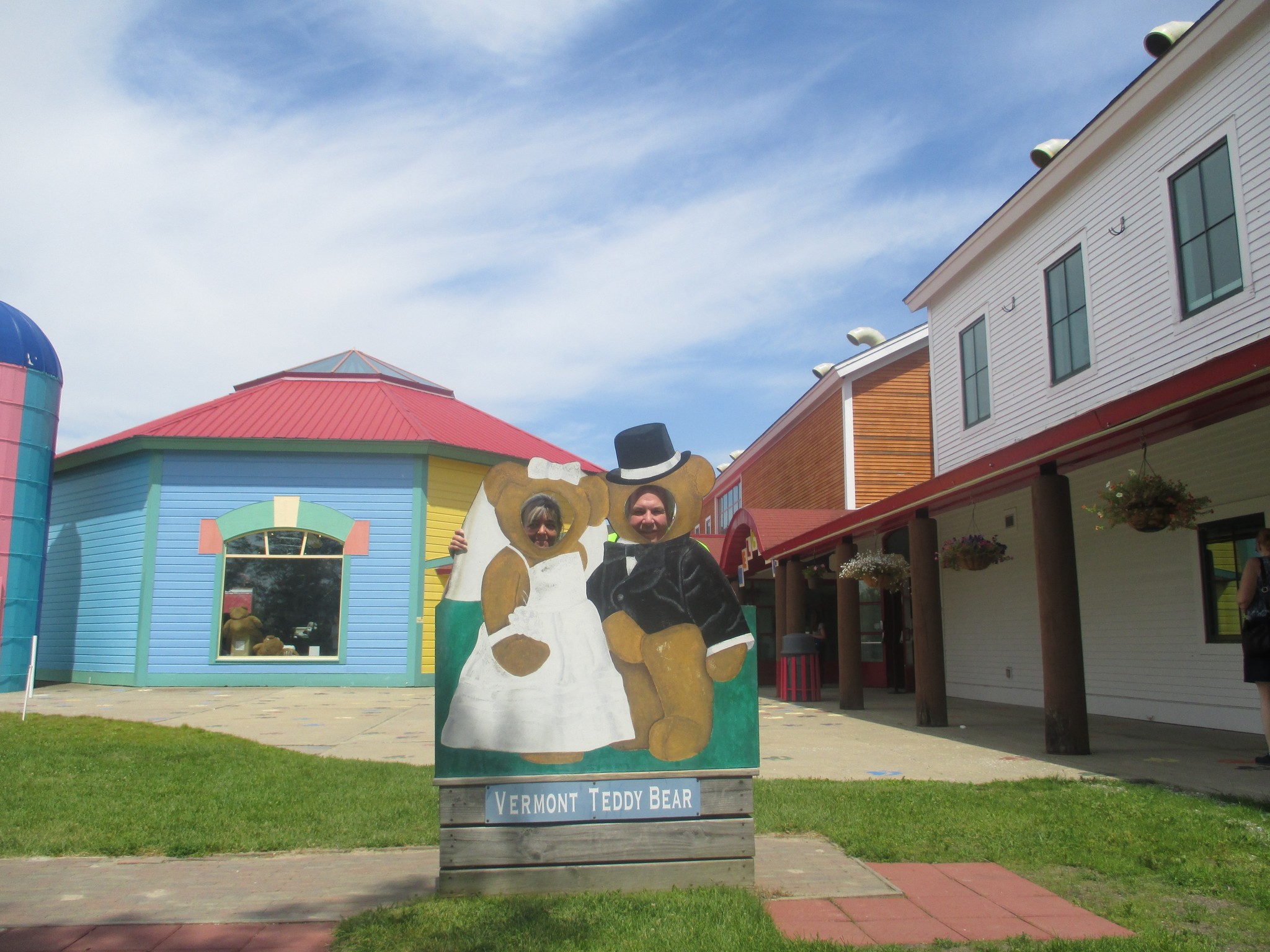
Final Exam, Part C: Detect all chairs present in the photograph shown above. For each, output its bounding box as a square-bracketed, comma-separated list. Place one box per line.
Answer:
[277, 648, 300, 655]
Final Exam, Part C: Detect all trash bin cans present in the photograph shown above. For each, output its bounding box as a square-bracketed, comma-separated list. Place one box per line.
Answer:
[779, 633, 818, 702]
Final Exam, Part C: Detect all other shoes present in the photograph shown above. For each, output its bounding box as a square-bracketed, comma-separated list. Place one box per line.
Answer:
[1255, 751, 1270, 765]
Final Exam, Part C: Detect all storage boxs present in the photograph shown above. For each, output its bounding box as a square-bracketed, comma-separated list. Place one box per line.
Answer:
[230, 635, 254, 656]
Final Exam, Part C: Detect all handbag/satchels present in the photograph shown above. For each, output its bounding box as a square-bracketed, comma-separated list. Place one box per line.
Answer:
[1239, 556, 1270, 621]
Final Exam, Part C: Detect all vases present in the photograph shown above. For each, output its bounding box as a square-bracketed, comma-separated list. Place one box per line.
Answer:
[807, 576, 821, 590]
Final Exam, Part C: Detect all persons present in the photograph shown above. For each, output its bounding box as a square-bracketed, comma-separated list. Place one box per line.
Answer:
[806, 608, 826, 655]
[448, 494, 563, 560]
[626, 485, 675, 542]
[1236, 528, 1270, 765]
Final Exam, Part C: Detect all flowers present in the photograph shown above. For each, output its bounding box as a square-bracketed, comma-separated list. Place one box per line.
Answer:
[840, 550, 914, 593]
[803, 563, 827, 579]
[934, 535, 1014, 572]
[1083, 469, 1216, 533]
